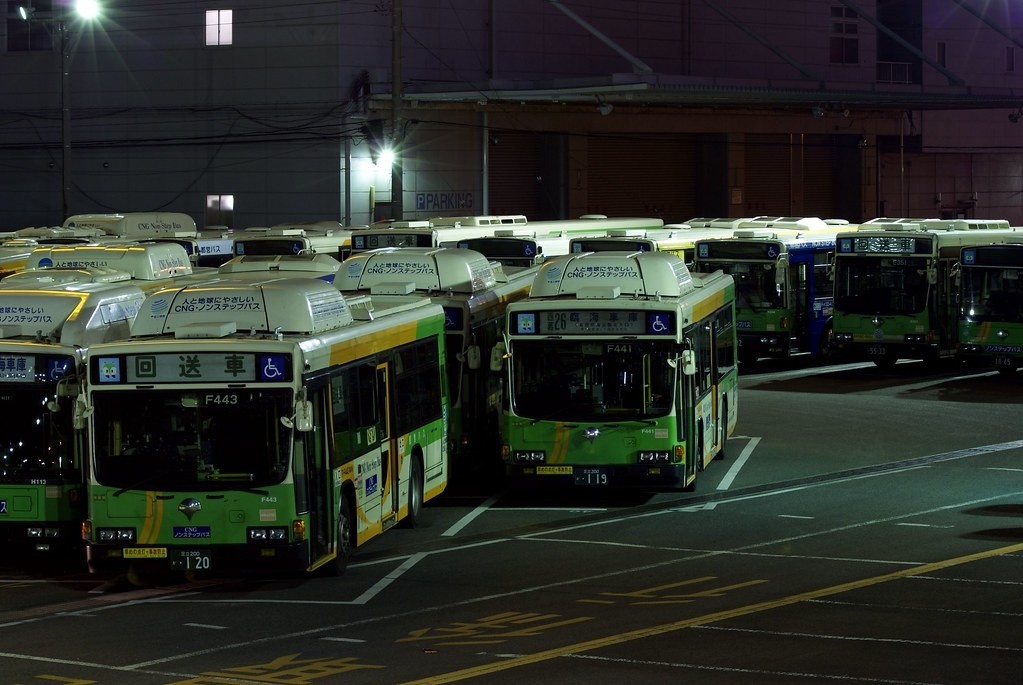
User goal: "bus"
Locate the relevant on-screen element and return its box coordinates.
[494,248,738,496]
[0,208,1023,571]
[70,270,455,578]
